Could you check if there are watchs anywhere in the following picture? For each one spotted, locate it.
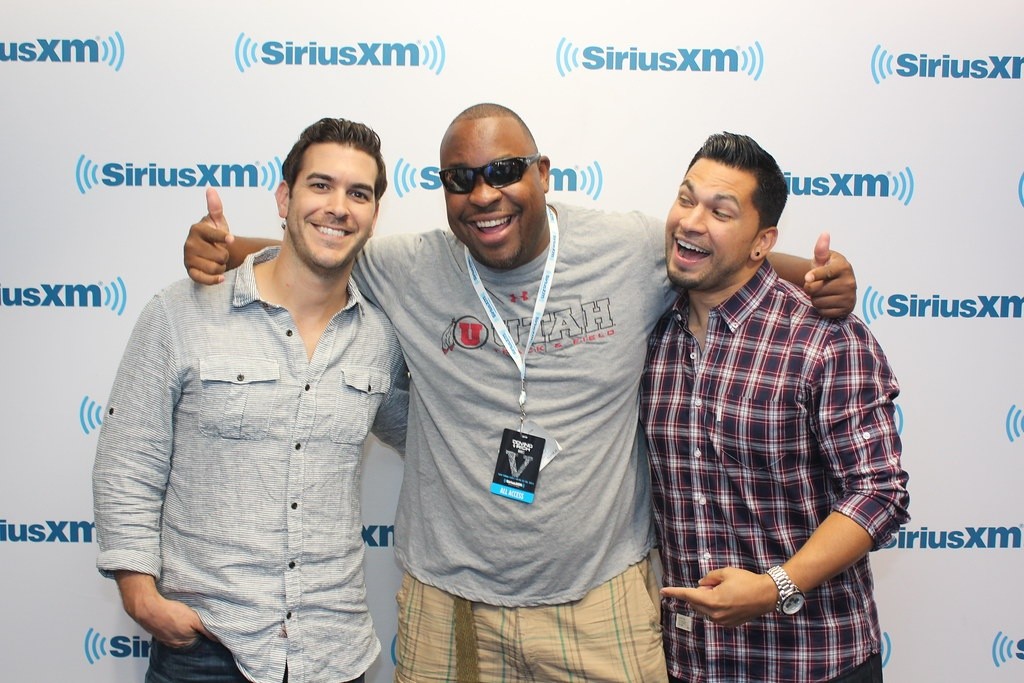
[767,565,805,616]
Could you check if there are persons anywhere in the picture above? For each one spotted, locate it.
[183,102,856,683]
[638,133,912,683]
[93,117,411,683]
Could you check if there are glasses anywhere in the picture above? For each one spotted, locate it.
[439,152,543,194]
